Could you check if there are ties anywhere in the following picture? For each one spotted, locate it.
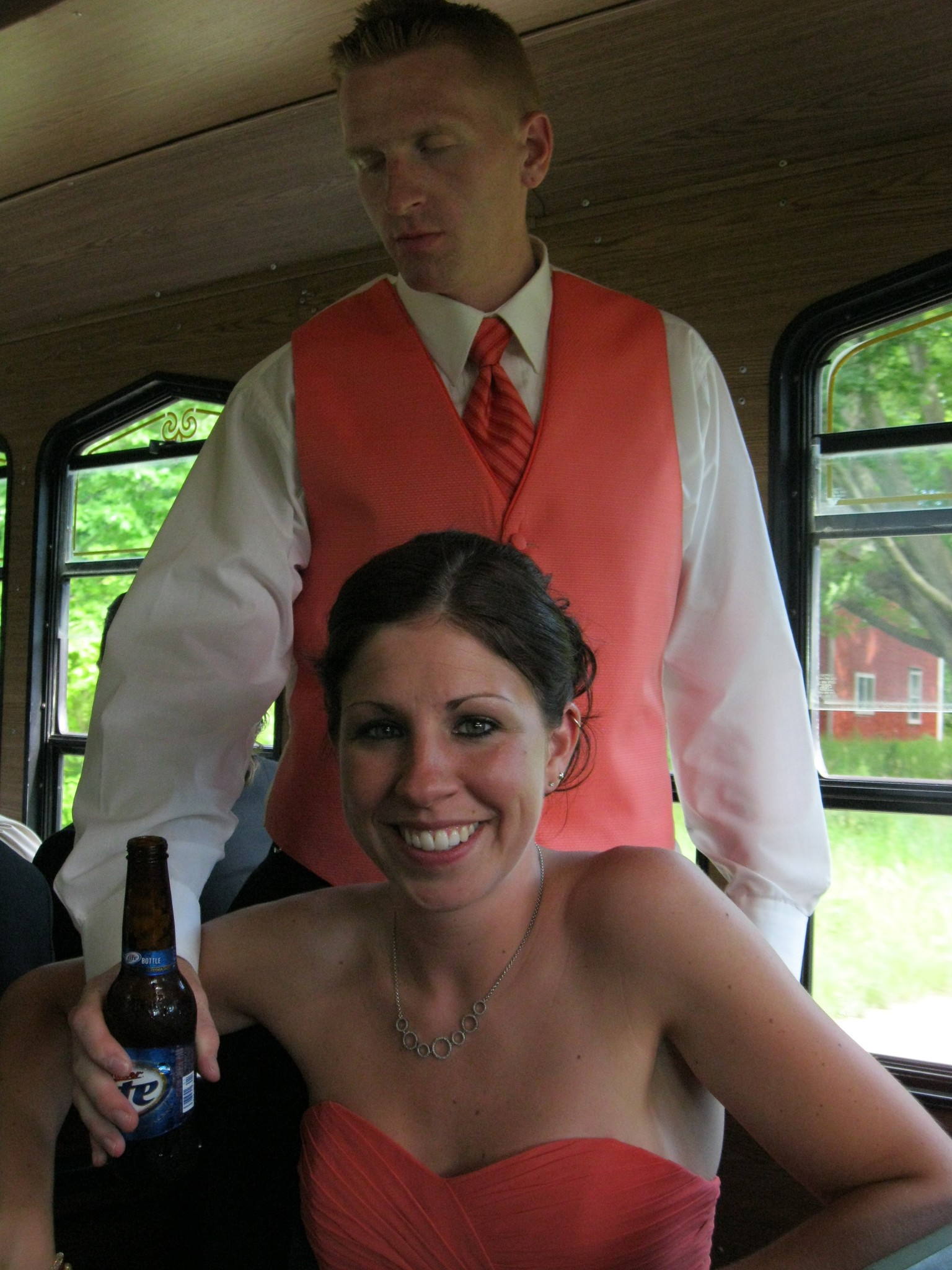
[461,318,538,502]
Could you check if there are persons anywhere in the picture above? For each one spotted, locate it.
[1,524,952,1270]
[47,1,828,1165]
[94,594,283,937]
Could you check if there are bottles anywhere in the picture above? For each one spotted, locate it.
[100,836,200,1174]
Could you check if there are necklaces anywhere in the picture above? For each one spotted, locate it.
[391,840,545,1061]
